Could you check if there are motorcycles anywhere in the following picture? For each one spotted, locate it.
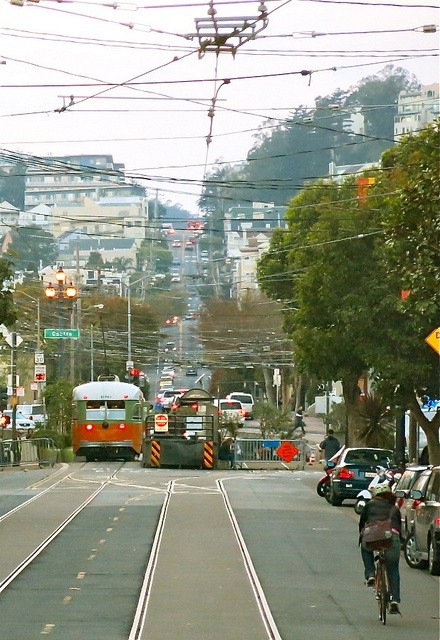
[317,460,336,497]
[354,453,403,515]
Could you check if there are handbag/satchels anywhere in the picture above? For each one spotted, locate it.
[361,520,395,551]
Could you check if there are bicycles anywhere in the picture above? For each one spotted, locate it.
[368,551,403,625]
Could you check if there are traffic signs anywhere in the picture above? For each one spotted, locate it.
[44,329,80,339]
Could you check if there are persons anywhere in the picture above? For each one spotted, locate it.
[288,394,296,411]
[218,438,237,470]
[26,428,33,439]
[359,484,401,615]
[319,429,340,464]
[292,403,306,434]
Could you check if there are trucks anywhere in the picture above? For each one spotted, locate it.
[161,223,172,234]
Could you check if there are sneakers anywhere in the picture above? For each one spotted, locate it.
[388,601,398,614]
[366,573,376,587]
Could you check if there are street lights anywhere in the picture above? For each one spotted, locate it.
[127,274,166,383]
[46,267,77,432]
[77,304,105,385]
[2,288,40,404]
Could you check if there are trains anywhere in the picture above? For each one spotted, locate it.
[73,374,152,461]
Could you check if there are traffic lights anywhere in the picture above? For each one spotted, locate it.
[129,369,139,380]
[1,416,10,428]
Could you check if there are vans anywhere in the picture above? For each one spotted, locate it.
[16,404,48,426]
[160,389,190,410]
[226,392,254,420]
[214,399,244,429]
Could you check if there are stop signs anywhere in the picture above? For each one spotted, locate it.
[154,414,168,433]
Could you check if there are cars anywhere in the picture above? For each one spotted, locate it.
[186,370,197,375]
[172,240,181,247]
[157,388,174,403]
[393,466,432,550]
[159,376,173,388]
[165,342,177,353]
[404,466,440,576]
[125,372,150,398]
[173,258,180,264]
[171,273,181,282]
[161,369,175,376]
[1,410,37,430]
[185,242,193,249]
[186,313,196,320]
[192,231,199,237]
[327,448,404,506]
[200,250,208,256]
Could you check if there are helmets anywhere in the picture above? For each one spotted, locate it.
[373,483,391,497]
